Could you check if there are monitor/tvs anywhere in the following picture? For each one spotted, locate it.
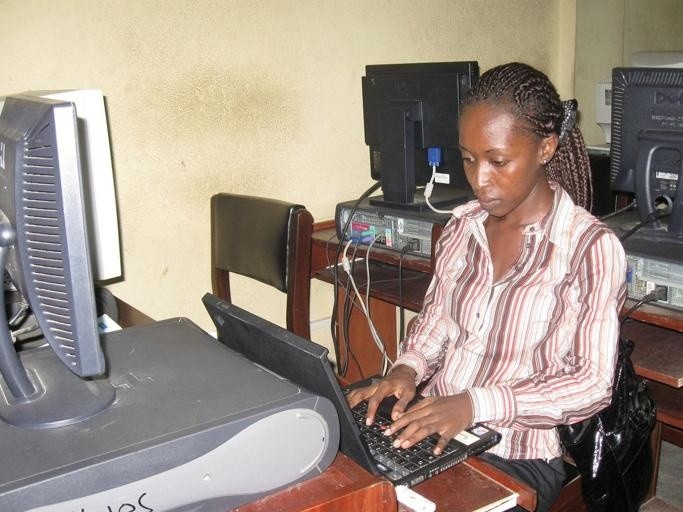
[361,61,482,214]
[608,67,683,244]
[0,93,116,428]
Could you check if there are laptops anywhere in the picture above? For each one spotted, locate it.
[201,292,503,487]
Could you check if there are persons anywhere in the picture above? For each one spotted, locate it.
[343,62,627,511]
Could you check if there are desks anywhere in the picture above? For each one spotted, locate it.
[1,283,540,512]
[309,220,682,450]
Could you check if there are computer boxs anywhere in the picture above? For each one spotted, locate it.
[0,315,340,511]
[601,210,683,315]
[334,193,474,261]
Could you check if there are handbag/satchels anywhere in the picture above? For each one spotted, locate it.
[580,340,657,512]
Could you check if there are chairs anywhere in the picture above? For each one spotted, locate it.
[210,193,314,342]
